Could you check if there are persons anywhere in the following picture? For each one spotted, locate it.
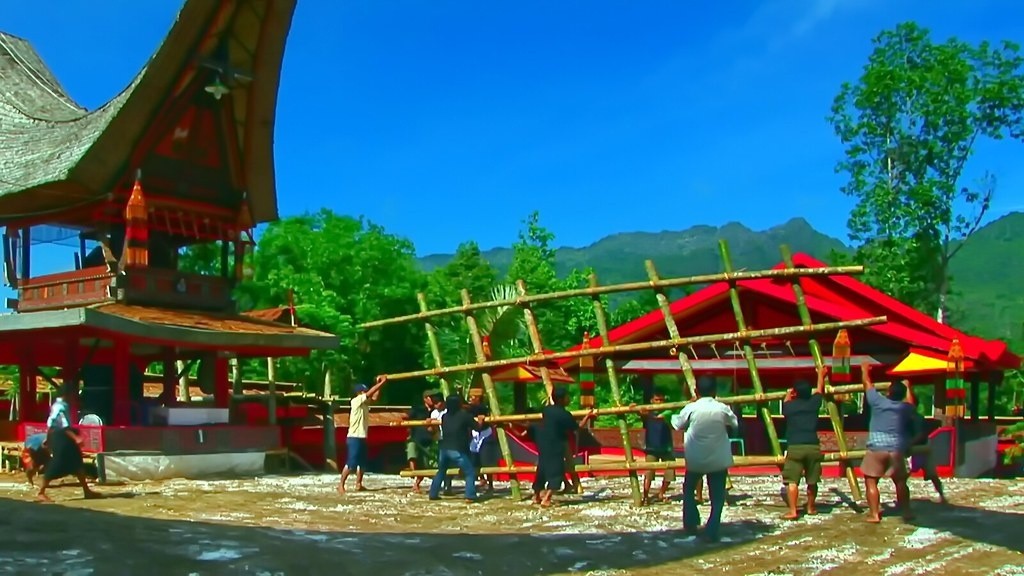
[861,360,949,524]
[629,391,677,507]
[337,374,387,493]
[780,364,829,518]
[38,383,103,503]
[531,388,599,505]
[671,377,738,540]
[395,388,495,501]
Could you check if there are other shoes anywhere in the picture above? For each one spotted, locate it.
[477,479,487,486]
[355,486,366,491]
[542,503,554,508]
[533,498,542,504]
[641,496,649,506]
[336,486,345,493]
[432,497,441,500]
[654,493,672,503]
[412,487,422,494]
[465,498,475,503]
[84,492,102,499]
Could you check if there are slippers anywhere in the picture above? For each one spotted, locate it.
[807,511,818,515]
[778,512,797,521]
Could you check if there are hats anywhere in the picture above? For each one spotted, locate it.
[423,390,431,397]
[469,388,484,396]
[353,384,368,392]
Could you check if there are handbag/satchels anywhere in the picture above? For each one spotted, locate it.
[36,493,55,502]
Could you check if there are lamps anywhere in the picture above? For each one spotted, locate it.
[203,73,229,98]
